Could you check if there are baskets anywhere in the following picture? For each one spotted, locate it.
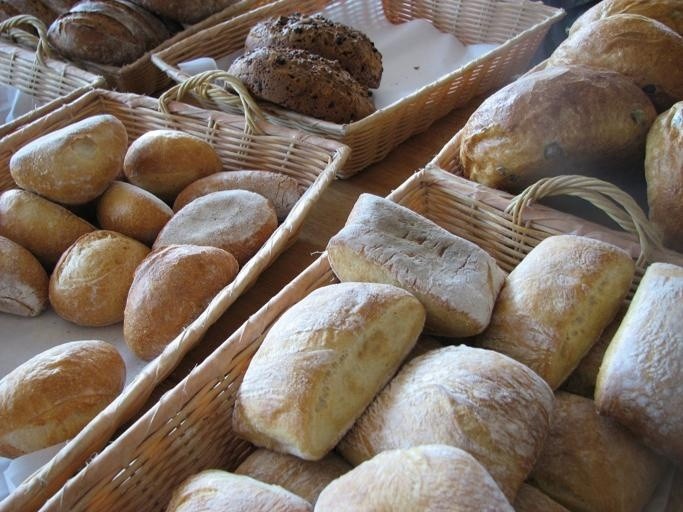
[423,60,682,266]
[0,45,105,137]
[1,1,683,511]
[37,166,678,510]
[0,69,353,511]
[151,0,568,182]
[0,0,255,98]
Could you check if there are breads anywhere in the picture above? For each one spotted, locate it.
[244,12,384,89]
[0,0,239,68]
[0,340,126,458]
[161,191,682,512]
[224,47,375,126]
[0,113,302,359]
[461,0,683,249]
